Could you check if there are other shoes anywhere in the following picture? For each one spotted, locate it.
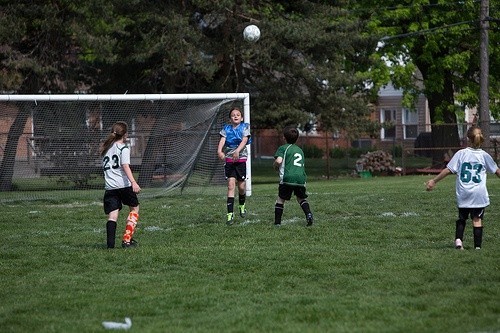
[455,239,463,248]
[475,247,481,250]
[121,238,138,248]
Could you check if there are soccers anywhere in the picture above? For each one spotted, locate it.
[243,24,261,42]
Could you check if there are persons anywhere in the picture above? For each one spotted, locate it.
[424,126,500,250]
[100,121,143,249]
[273,126,313,226]
[217,107,252,226]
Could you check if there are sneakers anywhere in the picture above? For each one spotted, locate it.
[239,204,249,220]
[305,213,314,227]
[226,213,235,226]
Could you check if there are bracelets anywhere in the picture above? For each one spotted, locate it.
[131,180,135,183]
